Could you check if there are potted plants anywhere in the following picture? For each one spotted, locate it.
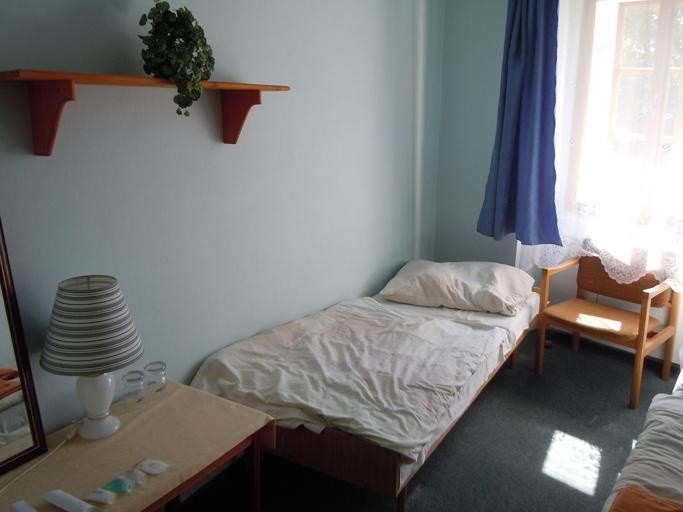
[135,1,215,115]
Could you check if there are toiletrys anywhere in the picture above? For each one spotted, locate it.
[83,468,146,505]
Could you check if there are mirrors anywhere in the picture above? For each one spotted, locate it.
[0,217,49,476]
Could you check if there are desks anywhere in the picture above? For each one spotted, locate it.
[0,380,281,512]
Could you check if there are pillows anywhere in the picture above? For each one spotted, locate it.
[378,255,535,317]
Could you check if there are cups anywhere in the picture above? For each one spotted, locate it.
[143,361,168,395]
[121,370,146,406]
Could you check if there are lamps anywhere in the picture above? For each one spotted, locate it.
[35,275,144,442]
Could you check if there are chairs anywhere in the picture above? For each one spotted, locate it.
[532,234,679,409]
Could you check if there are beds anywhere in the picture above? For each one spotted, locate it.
[189,286,545,511]
[599,372,682,511]
